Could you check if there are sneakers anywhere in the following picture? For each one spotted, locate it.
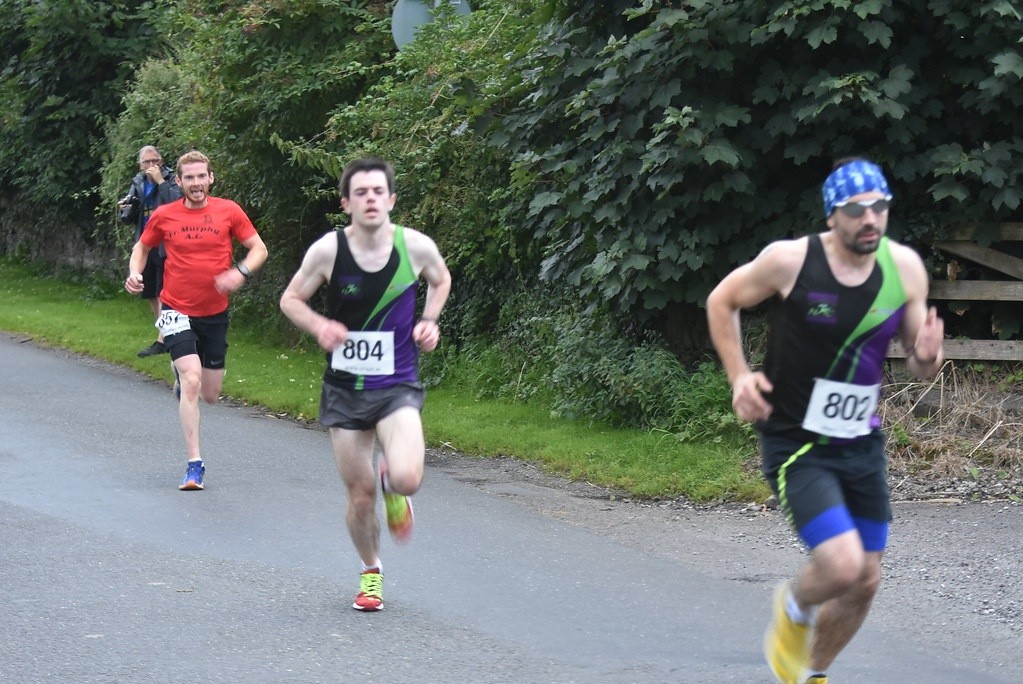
[353,563,385,612]
[798,667,829,684]
[171,363,182,401]
[177,460,205,490]
[374,455,414,542]
[764,580,808,684]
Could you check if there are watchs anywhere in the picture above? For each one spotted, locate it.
[235,263,254,284]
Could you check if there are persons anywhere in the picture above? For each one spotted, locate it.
[122,149,268,491]
[280,158,453,613]
[704,156,947,684]
[118,145,184,357]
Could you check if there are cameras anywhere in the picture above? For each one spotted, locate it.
[121,195,141,225]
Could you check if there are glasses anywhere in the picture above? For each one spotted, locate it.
[140,158,161,165]
[831,197,891,217]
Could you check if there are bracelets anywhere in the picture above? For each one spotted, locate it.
[910,344,939,367]
[420,317,435,323]
